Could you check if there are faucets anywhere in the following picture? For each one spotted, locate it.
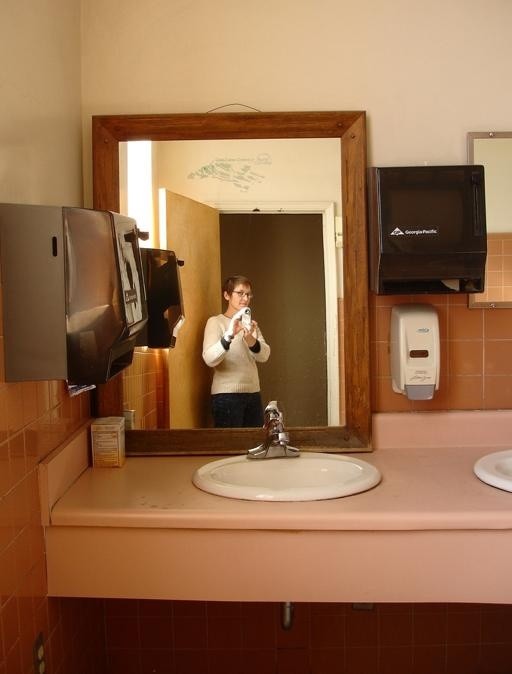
[246,402,299,459]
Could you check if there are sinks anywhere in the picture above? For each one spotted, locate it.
[474,449,512,493]
[191,452,382,502]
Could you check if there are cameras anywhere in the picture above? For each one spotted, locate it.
[242,307,251,328]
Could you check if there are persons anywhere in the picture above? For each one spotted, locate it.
[202,274,272,427]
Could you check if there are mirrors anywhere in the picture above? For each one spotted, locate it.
[467,131,512,308]
[91,111,373,457]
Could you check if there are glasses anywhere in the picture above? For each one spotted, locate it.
[232,291,253,298]
